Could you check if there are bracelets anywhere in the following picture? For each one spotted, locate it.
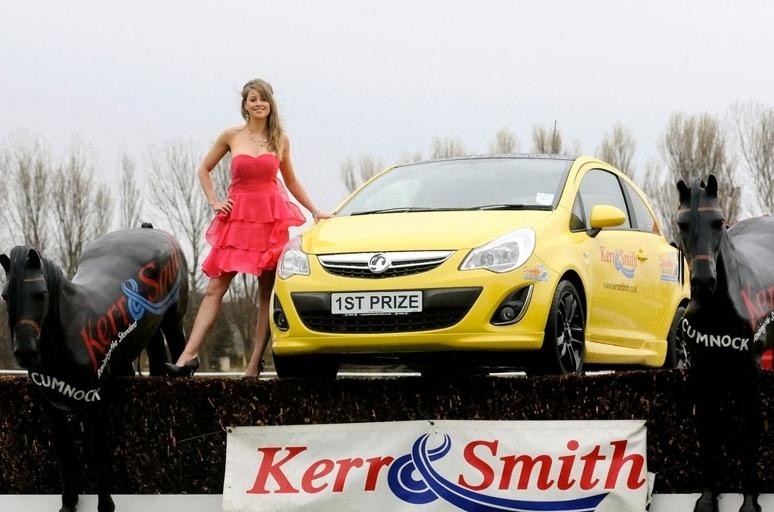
[312,209,320,217]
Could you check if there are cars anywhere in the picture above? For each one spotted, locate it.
[266,154,687,379]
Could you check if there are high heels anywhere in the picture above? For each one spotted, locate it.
[239,359,265,382]
[164,354,200,379]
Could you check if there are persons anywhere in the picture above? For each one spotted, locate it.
[165,79,332,381]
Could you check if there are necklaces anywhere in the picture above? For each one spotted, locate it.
[248,130,267,147]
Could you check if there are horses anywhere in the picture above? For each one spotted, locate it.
[672,172,774,512]
[0,221,191,512]
